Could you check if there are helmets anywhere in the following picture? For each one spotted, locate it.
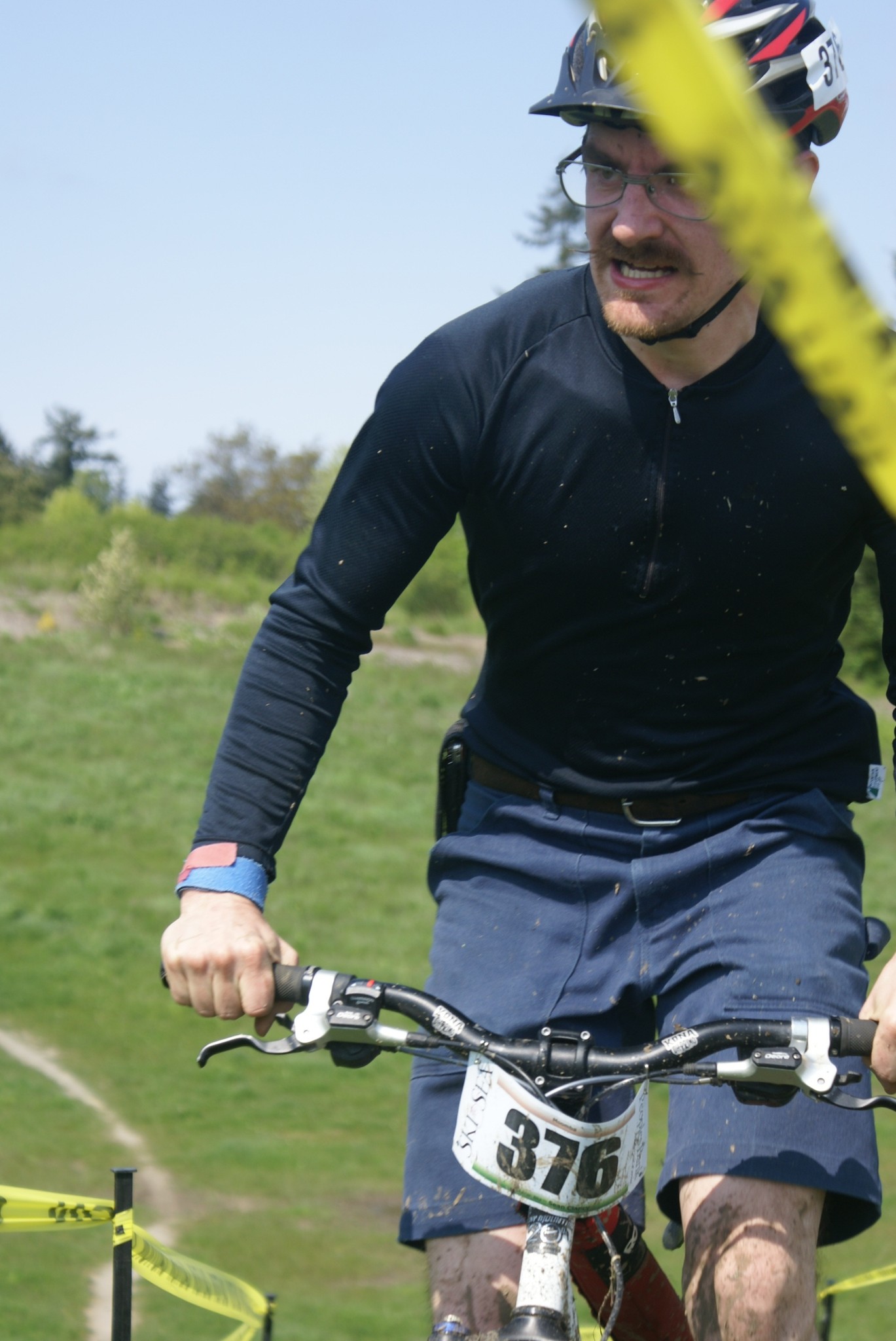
[527,0,851,146]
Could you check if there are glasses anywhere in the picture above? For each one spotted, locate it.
[554,149,724,221]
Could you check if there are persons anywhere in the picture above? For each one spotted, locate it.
[158,0,896,1341]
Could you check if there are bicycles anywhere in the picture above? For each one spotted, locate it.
[161,959,896,1341]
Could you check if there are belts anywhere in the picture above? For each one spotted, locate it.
[463,752,757,829]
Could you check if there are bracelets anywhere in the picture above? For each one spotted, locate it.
[175,842,268,912]
